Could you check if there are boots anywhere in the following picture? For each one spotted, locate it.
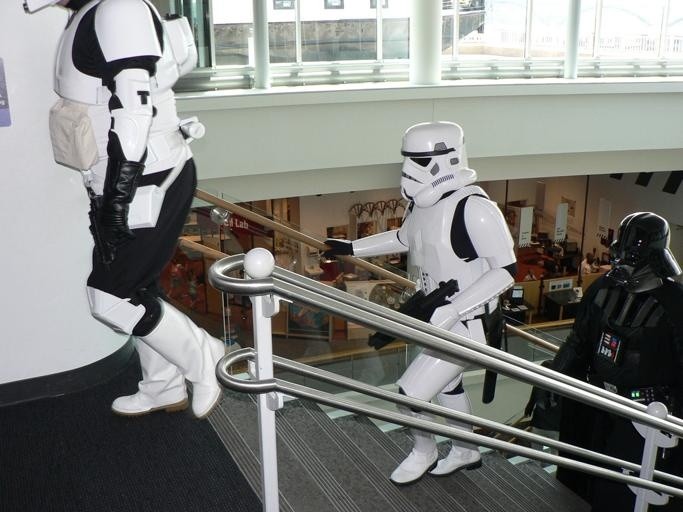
[389,392,481,485]
[111,297,225,419]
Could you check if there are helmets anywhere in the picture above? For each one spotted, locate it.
[606,212,682,293]
[401,122,476,207]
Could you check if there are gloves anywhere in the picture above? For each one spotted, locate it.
[100,161,145,244]
[319,239,353,260]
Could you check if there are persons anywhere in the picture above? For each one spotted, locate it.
[17,0,226,424]
[185,268,200,312]
[523,358,567,467]
[523,269,538,281]
[583,256,593,274]
[320,118,518,487]
[165,258,188,300]
[542,210,682,511]
[576,251,592,281]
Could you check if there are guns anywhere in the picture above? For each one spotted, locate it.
[368,279,459,351]
[90,193,126,269]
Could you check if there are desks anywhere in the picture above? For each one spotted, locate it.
[542,289,582,321]
[505,297,535,323]
